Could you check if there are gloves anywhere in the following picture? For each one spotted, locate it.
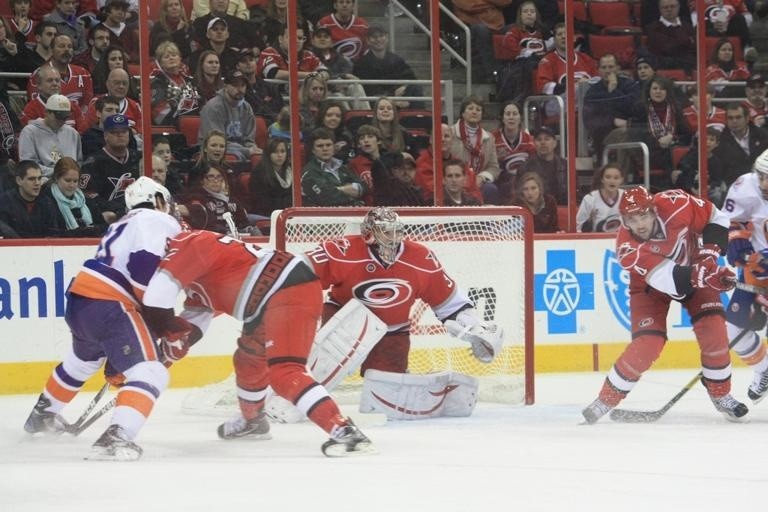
[152,318,194,361]
[690,221,768,291]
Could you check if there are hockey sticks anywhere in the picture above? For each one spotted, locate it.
[612,310,757,424]
[57,358,169,436]
[54,382,109,430]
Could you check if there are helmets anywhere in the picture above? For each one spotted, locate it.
[124,176,171,211]
[755,149,768,175]
[620,186,656,229]
[360,206,404,264]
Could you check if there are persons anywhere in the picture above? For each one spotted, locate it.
[295,206,506,416]
[717,147,767,400]
[0,2,768,237]
[579,186,749,420]
[20,175,184,462]
[103,222,375,456]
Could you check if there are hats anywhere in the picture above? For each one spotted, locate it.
[226,70,248,83]
[46,95,71,118]
[207,17,228,31]
[104,114,127,129]
